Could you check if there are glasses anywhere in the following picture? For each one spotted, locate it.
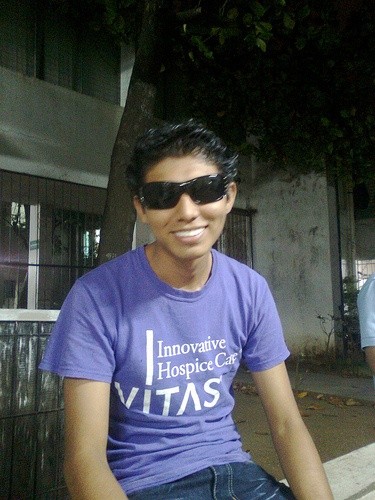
[139,170,229,211]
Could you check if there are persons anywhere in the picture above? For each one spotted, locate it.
[38,120,340,500]
[356,270,375,381]
